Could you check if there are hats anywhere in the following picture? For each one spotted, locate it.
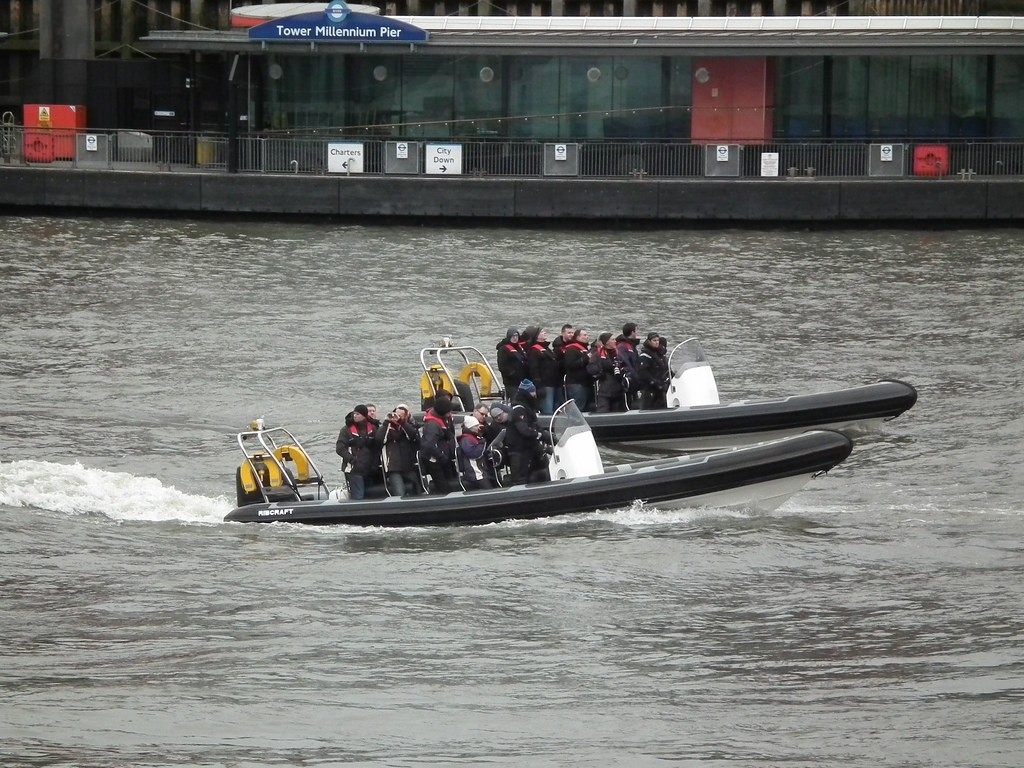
[519,379,535,393]
[354,404,368,418]
[599,333,613,345]
[529,327,543,340]
[491,407,503,418]
[507,328,519,339]
[464,416,479,429]
[434,398,451,415]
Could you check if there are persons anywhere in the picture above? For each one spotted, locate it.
[494,320,680,419]
[335,378,559,499]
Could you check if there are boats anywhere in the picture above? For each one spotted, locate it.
[411,336,917,449]
[224,398,853,527]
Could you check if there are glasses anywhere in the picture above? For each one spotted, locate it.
[477,410,488,417]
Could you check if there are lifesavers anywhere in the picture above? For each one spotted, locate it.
[459,362,493,397]
[421,364,455,399]
[271,444,312,483]
[240,454,283,494]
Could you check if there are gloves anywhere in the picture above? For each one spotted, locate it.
[537,388,546,399]
[439,454,448,465]
[479,438,486,445]
[350,457,356,464]
